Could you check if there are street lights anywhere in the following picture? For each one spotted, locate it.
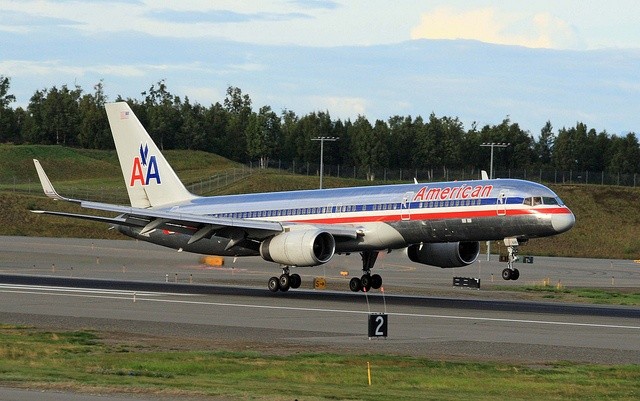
[309,133,343,189]
[480,138,511,180]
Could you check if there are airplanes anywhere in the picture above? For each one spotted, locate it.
[25,99,578,295]
